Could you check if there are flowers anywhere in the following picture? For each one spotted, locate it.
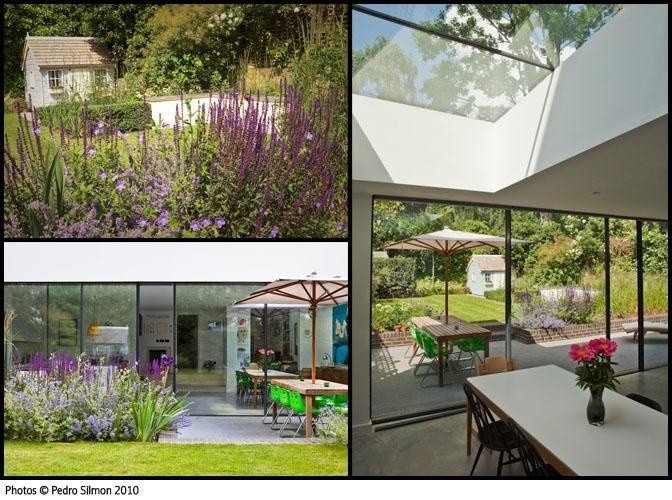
[568,338,621,398]
[255,347,275,368]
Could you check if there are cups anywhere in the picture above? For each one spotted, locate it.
[455,325,459,329]
[324,382,329,387]
[300,376,304,381]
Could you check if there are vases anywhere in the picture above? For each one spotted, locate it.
[262,362,268,373]
[587,385,605,426]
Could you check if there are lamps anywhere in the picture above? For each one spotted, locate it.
[86,283,100,337]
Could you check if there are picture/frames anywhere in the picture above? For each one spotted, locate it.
[59,319,77,347]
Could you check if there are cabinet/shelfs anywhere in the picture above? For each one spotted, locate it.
[301,368,348,385]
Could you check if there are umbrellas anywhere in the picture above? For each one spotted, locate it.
[234,272,348,384]
[379,225,534,324]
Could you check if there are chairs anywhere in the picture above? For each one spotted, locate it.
[405,314,491,387]
[477,356,518,377]
[625,393,661,413]
[505,417,563,477]
[462,383,537,476]
[236,367,349,439]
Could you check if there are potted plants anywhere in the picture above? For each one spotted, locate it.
[203,360,216,374]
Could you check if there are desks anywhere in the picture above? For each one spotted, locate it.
[88,361,121,390]
[465,365,669,476]
[15,367,48,385]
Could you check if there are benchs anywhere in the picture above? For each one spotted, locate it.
[622,321,668,343]
